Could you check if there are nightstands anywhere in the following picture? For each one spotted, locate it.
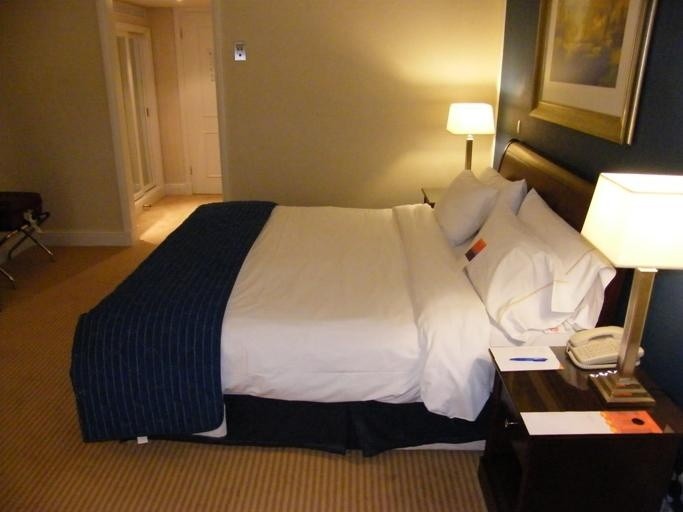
[421,186,446,208]
[478,349,683,512]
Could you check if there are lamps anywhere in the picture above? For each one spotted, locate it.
[580,173,683,409]
[446,102,497,169]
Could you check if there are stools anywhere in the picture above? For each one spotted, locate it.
[0,192,57,288]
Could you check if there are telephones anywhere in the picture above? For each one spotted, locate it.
[565,326,645,370]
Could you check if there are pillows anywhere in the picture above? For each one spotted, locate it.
[433,167,617,347]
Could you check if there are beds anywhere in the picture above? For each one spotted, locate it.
[70,138,635,457]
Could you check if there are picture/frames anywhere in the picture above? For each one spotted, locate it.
[528,0,659,147]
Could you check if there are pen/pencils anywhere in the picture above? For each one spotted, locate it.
[510,358,548,362]
